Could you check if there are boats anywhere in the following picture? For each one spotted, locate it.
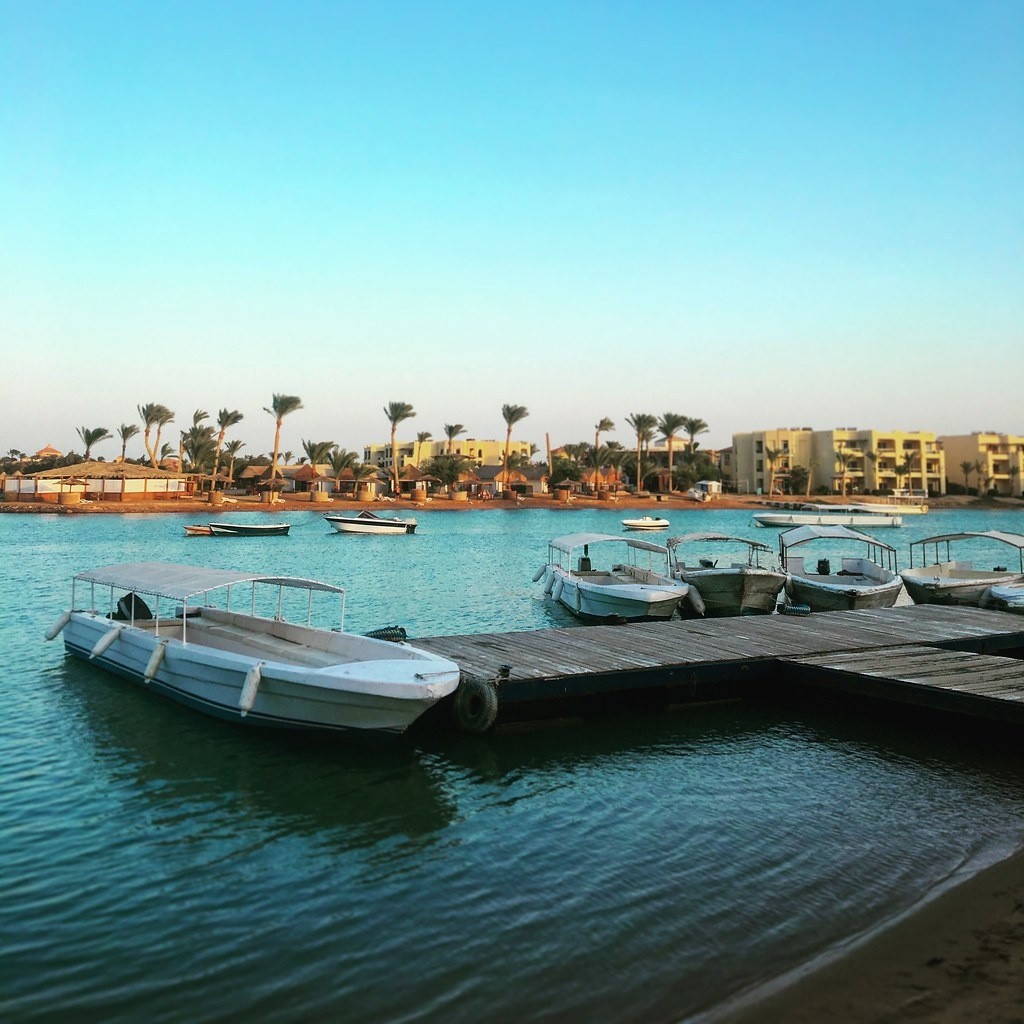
[666,533,785,617]
[850,495,929,514]
[60,561,460,738]
[323,510,417,535]
[978,580,1024,614]
[752,504,903,528]
[899,530,1024,607]
[182,525,212,535]
[543,532,690,623]
[207,522,291,536]
[620,518,669,530]
[778,523,904,611]
